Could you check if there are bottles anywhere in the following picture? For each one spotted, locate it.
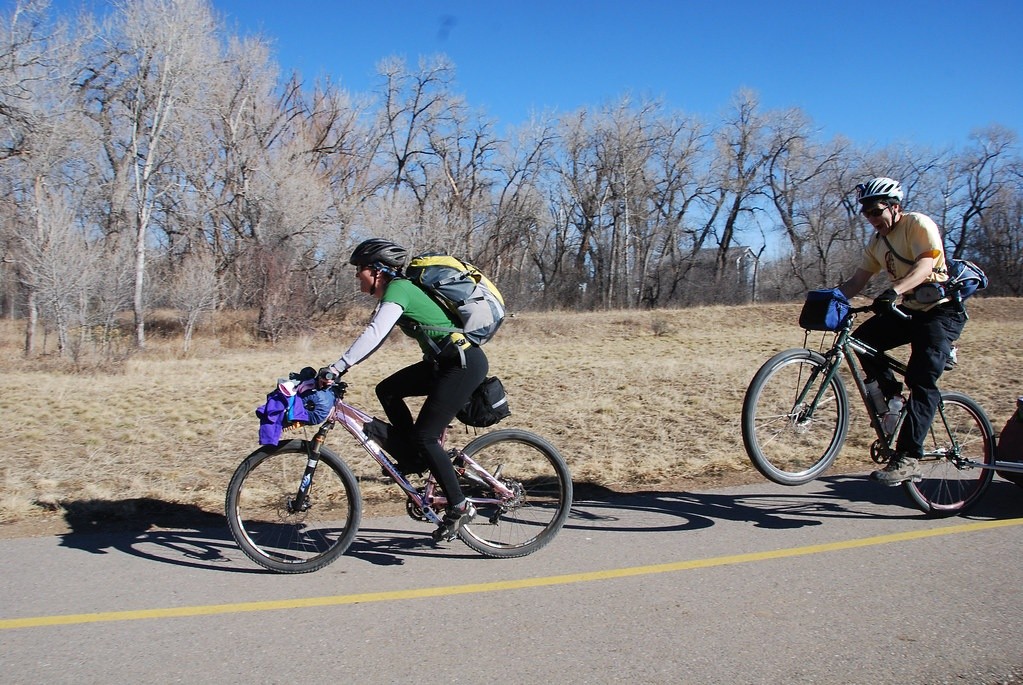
[863,377,889,415]
[880,394,904,436]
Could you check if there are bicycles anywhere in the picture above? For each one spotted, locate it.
[739,287,997,518]
[224,366,574,575]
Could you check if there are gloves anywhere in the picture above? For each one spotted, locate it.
[872,288,898,317]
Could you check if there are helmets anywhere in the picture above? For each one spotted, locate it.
[349,237,408,268]
[855,177,904,201]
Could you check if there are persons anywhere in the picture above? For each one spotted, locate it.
[314,238,489,539]
[837,177,964,485]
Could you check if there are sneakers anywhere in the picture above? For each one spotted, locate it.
[432,500,478,539]
[869,456,922,486]
[382,464,420,476]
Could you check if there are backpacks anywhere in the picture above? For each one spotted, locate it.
[405,246,505,347]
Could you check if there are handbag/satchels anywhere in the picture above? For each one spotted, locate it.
[945,256,989,299]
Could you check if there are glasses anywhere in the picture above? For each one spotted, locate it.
[356,265,366,274]
[862,206,890,218]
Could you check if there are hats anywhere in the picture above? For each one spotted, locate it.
[858,195,902,216]
